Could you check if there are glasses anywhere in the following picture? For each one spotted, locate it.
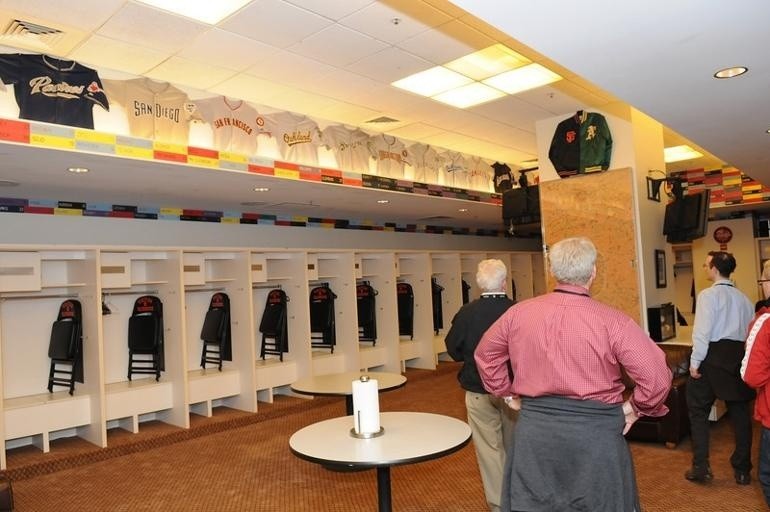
[702,263,714,267]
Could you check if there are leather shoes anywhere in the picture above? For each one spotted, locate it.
[685,466,711,481]
[736,470,751,484]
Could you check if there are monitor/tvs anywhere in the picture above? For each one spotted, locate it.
[662,189,711,244]
[647,303,676,343]
[502,185,541,225]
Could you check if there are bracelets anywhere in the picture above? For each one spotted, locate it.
[504,396,513,404]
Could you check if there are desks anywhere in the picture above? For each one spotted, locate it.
[287,370,473,510]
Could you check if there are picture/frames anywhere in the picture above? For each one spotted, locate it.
[653,249,667,288]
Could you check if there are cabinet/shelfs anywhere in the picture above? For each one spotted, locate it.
[0,241,543,451]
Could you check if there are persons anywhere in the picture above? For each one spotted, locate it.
[444,257,520,511]
[740,260,770,510]
[474,236,673,512]
[683,250,754,483]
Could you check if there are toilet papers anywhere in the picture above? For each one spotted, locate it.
[351,378,381,435]
